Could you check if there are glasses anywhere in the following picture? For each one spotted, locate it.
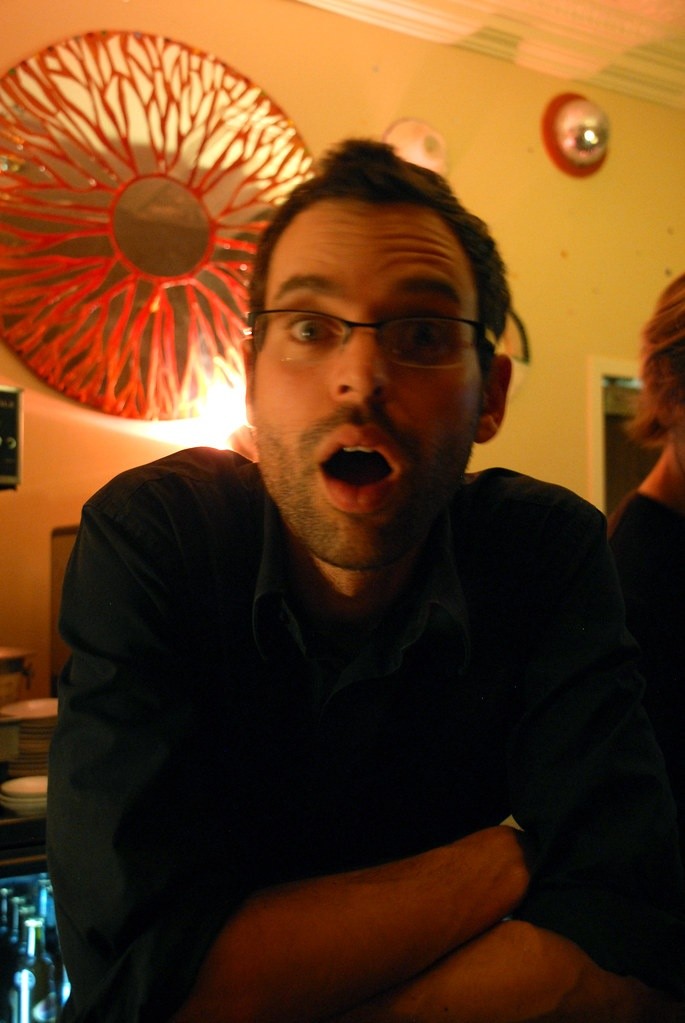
[247,308,488,368]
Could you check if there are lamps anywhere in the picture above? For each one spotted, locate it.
[380,118,453,182]
[539,92,609,179]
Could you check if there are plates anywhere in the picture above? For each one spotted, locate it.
[0,776,49,818]
[0,698,58,775]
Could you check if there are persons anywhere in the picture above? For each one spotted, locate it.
[46,137,685,1023]
[605,271,684,858]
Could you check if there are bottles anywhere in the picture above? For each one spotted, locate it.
[0,873,65,1023]
